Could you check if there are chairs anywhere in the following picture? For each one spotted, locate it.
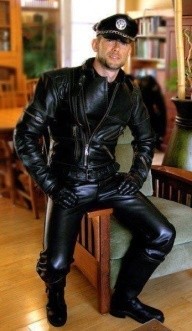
[0,75,48,219]
[73,126,192,313]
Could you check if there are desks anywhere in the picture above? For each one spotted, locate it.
[0,106,23,141]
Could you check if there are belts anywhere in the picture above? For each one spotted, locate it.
[66,163,120,183]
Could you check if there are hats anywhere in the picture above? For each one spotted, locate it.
[93,13,139,43]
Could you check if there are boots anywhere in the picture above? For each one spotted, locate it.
[45,277,67,327]
[110,252,165,324]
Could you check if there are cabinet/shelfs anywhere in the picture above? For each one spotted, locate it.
[0,0,23,93]
[128,16,192,99]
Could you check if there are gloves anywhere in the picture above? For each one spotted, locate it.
[118,171,143,195]
[50,184,80,209]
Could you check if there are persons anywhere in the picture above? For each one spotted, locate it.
[14,14,176,328]
[125,74,177,156]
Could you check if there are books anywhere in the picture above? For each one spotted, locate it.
[132,16,176,94]
